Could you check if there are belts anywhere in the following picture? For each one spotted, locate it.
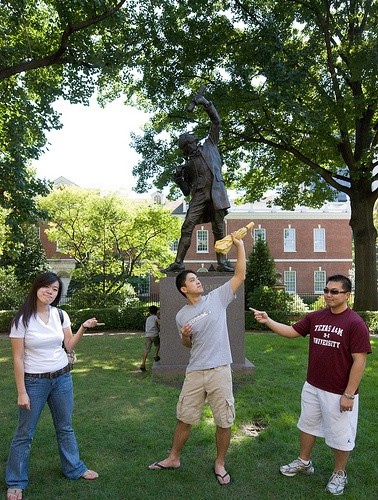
[25,364,71,379]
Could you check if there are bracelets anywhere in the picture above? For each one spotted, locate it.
[343,393,354,400]
[82,323,85,329]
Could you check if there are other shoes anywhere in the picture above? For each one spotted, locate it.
[139,365,146,371]
[154,356,160,361]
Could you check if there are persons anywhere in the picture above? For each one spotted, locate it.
[7,273,105,500]
[149,230,246,485]
[140,306,160,371]
[250,275,373,493]
[160,96,235,273]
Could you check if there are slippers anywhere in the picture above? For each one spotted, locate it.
[147,462,181,470]
[6,489,24,500]
[82,470,99,479]
[212,467,232,486]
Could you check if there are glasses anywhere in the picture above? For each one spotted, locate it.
[323,288,346,294]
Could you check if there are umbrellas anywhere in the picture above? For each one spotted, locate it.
[214,221,256,254]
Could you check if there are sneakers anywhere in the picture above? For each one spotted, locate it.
[325,470,348,495]
[279,459,315,477]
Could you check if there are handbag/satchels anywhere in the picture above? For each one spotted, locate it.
[58,309,77,365]
